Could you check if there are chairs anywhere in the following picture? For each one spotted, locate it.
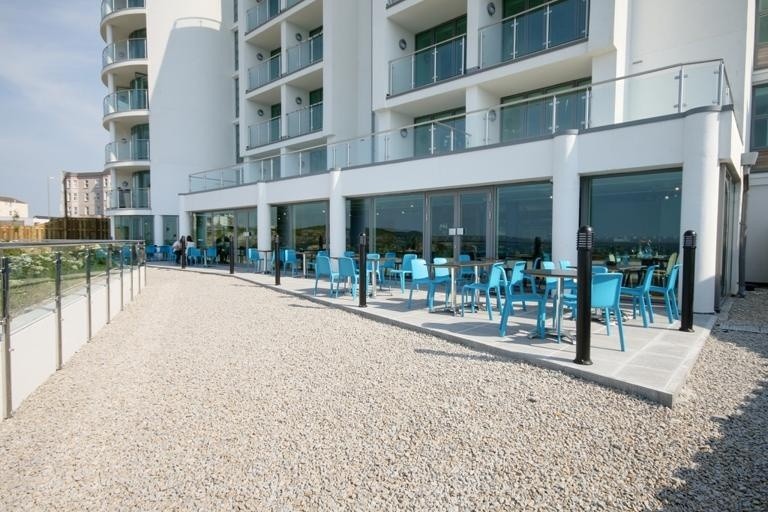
[495,253,628,354]
[144,242,223,269]
[632,263,684,324]
[247,243,313,278]
[314,247,527,319]
[604,264,660,327]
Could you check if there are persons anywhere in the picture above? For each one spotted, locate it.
[186,235,195,265]
[172,235,185,264]
[216,232,229,264]
